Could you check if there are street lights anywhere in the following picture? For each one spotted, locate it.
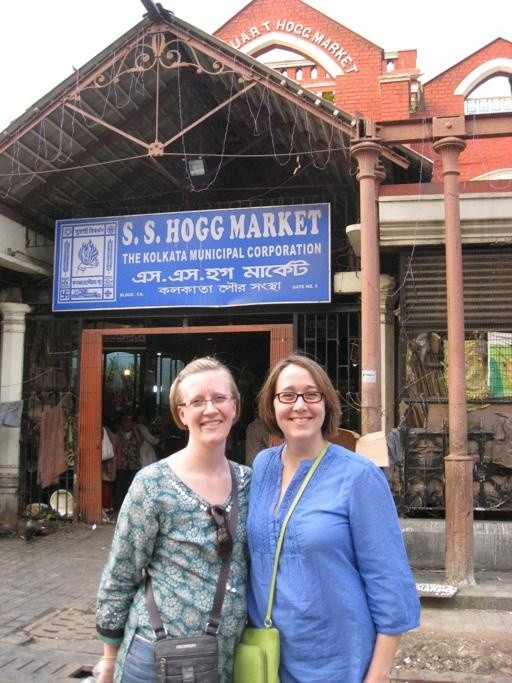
[230,623,283,682]
[154,633,222,683]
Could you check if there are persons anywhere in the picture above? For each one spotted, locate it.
[244,355,422,683]
[105,404,169,501]
[94,355,257,683]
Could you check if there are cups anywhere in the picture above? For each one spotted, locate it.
[173,393,240,411]
[274,390,325,404]
[210,503,234,561]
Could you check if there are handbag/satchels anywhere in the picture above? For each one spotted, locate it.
[102,654,117,659]
